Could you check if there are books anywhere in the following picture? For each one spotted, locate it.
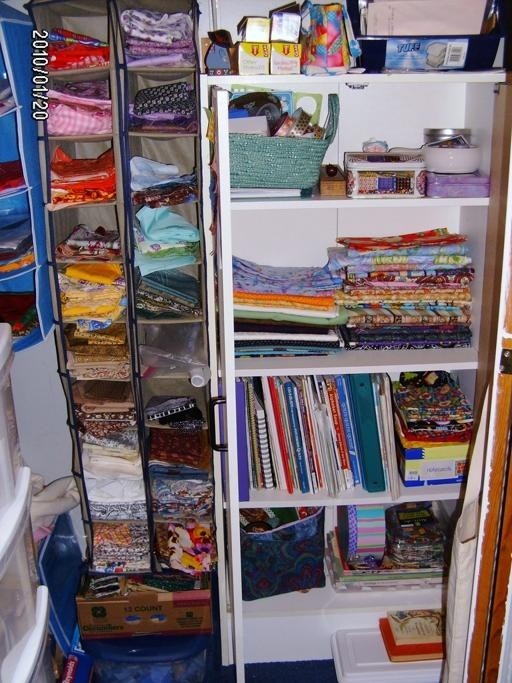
[234,374,403,504]
[377,609,452,667]
[324,527,445,591]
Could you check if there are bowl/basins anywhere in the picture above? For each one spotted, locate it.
[425,144,482,173]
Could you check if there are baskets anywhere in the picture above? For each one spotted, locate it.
[230,95,339,189]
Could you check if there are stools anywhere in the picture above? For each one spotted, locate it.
[81,630,213,682]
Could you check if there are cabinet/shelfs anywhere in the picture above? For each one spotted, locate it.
[22,0,227,585]
[195,68,512,683]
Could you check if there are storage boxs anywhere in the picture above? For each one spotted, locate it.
[427,166,490,198]
[390,386,474,489]
[240,507,327,605]
[1,321,51,681]
[359,0,495,37]
[77,571,213,641]
[341,151,425,200]
[355,35,503,73]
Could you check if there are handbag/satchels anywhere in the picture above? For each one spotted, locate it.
[300,0,362,76]
